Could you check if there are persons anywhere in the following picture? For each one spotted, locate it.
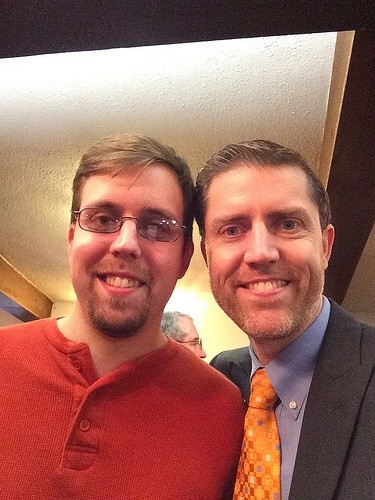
[160,312,206,361]
[0,133,245,500]
[194,139,375,500]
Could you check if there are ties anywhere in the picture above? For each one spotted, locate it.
[232,366,282,500]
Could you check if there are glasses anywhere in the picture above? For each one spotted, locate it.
[73,208,189,243]
[174,338,202,350]
